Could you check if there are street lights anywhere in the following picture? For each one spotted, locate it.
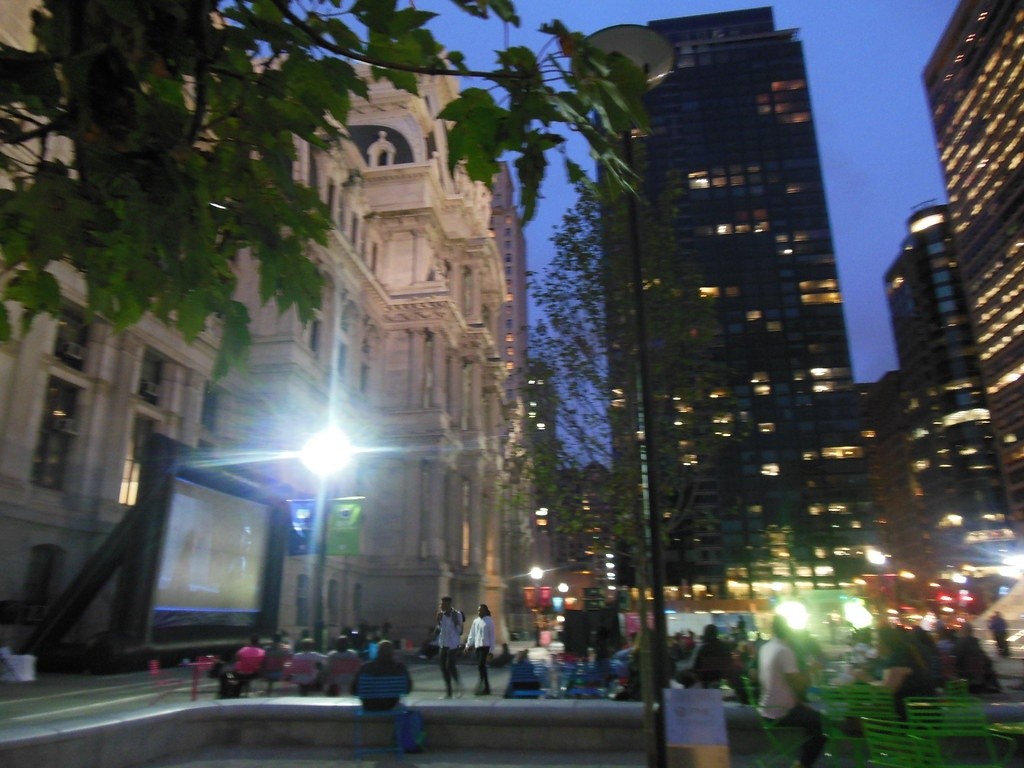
[572,23,679,768]
[298,426,359,648]
[530,563,544,613]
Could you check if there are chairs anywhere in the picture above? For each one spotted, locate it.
[351,674,409,762]
[743,674,1024,768]
[511,651,625,701]
[149,655,363,707]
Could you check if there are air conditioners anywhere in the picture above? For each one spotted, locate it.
[143,381,161,399]
[63,341,84,360]
[55,418,82,438]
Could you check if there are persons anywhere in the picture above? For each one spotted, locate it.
[433,597,463,700]
[464,604,494,695]
[234,628,414,711]
[533,610,1012,768]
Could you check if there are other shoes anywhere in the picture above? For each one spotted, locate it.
[478,688,490,695]
[456,689,462,699]
[438,695,452,699]
